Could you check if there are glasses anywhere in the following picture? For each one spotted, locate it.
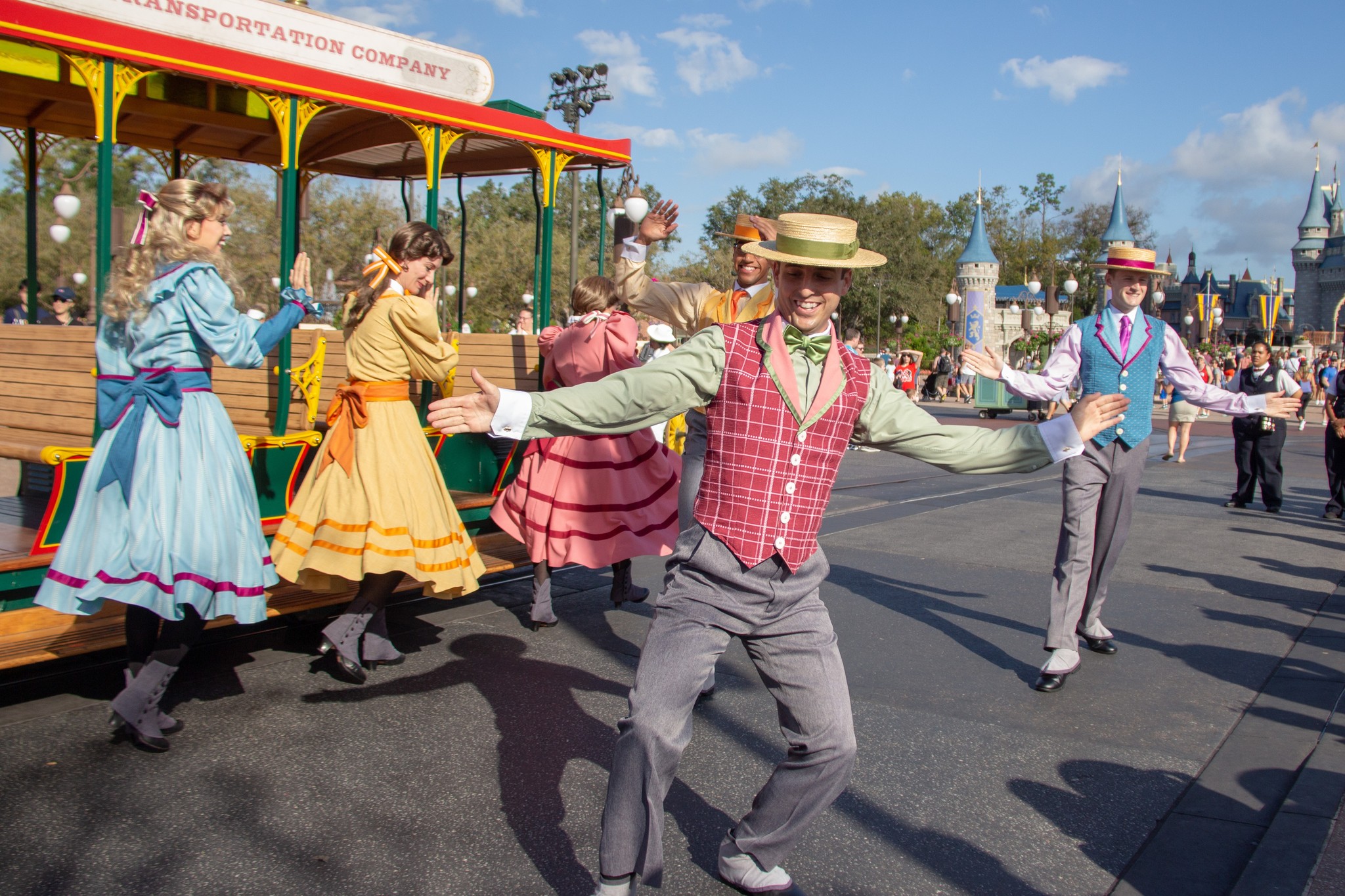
[734,242,763,259]
[902,354,911,357]
[518,316,533,321]
[52,295,69,304]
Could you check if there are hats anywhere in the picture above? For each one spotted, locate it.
[1290,352,1296,357]
[50,288,76,302]
[1196,354,1205,361]
[742,213,887,267]
[1089,246,1171,276]
[715,214,779,242]
[647,324,676,343]
[1331,356,1337,361]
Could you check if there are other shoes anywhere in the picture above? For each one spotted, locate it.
[1225,497,1343,518]
[938,394,973,404]
[1322,420,1328,425]
[1316,400,1326,406]
[1199,413,1208,418]
[719,872,807,896]
[1152,404,1168,409]
[1299,419,1305,431]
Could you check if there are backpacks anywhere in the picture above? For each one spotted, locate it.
[938,356,952,374]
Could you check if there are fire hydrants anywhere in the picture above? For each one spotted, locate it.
[544,61,615,318]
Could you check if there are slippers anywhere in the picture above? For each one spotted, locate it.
[1161,452,1187,463]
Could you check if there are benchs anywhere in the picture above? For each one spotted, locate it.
[0,323,547,558]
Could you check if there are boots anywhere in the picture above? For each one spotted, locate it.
[108,560,650,751]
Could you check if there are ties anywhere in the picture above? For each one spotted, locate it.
[731,289,748,323]
[1119,316,1132,364]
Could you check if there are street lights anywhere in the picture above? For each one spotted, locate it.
[832,301,842,341]
[1234,326,1239,356]
[1009,291,1043,371]
[1184,298,1224,353]
[50,157,100,326]
[1152,279,1165,320]
[946,277,962,369]
[890,304,909,359]
[606,164,649,313]
[1028,259,1078,359]
[1241,330,1247,346]
[521,278,534,309]
[444,269,477,327]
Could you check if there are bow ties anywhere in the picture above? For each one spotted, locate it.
[783,324,833,366]
[1253,368,1265,372]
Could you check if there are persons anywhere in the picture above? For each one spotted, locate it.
[5,279,51,324]
[873,348,923,405]
[614,200,779,699]
[490,276,683,631]
[507,308,540,335]
[32,178,325,750]
[1038,388,1074,423]
[638,324,676,366]
[1155,353,1197,464]
[962,248,1302,693]
[426,213,1130,896]
[267,221,487,680]
[1153,349,1251,418]
[843,328,864,358]
[1275,348,1345,430]
[1026,355,1041,370]
[932,342,976,404]
[1225,340,1303,513]
[1323,368,1345,519]
[40,287,83,325]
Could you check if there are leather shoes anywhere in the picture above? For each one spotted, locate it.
[1074,628,1118,654]
[1035,661,1081,692]
